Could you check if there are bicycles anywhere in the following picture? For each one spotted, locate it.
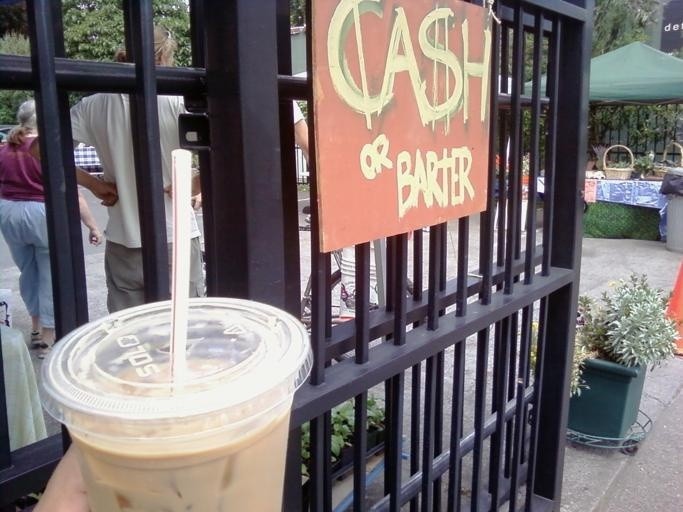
[201,205,417,364]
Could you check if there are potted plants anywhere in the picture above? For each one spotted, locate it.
[530,271,682,457]
[300,393,386,484]
[591,144,610,171]
[634,157,655,179]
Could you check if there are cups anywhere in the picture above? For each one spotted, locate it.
[37,294,314,512]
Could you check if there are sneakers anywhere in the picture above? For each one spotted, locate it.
[31,331,52,356]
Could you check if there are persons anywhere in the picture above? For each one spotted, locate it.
[191,191,203,210]
[290,91,310,172]
[29,438,96,512]
[28,27,206,315]
[585,143,598,170]
[0,99,106,359]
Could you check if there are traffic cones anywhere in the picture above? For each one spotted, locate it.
[663,260,683,358]
